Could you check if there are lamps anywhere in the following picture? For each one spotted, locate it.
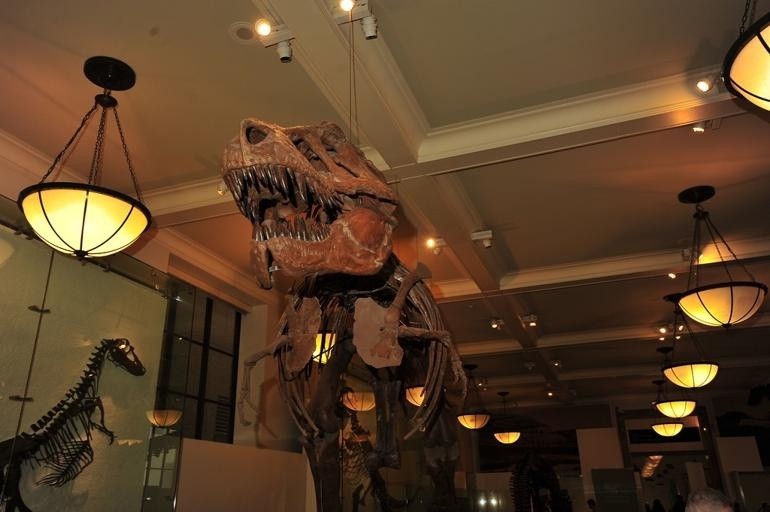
[651,185,768,437]
[334,0,378,40]
[457,367,491,429]
[340,356,375,413]
[723,1,769,114]
[407,357,448,408]
[697,72,722,96]
[489,319,502,330]
[427,237,441,256]
[144,408,182,427]
[529,316,536,327]
[693,118,721,133]
[642,454,664,478]
[17,56,152,258]
[480,230,493,248]
[255,17,294,63]
[310,314,338,366]
[494,390,521,444]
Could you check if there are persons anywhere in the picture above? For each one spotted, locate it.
[586,479,769,511]
